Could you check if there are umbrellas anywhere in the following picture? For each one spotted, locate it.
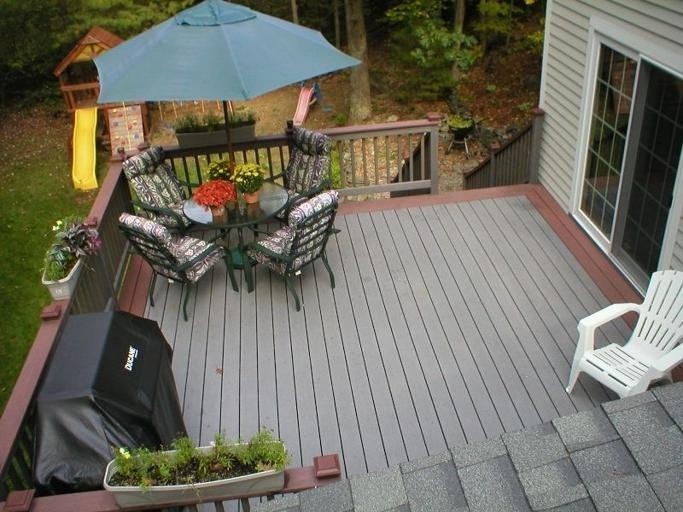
[92,0,361,208]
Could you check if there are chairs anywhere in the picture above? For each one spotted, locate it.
[252,128,333,235]
[243,190,340,312]
[122,146,227,240]
[118,212,238,321]
[565,270,683,399]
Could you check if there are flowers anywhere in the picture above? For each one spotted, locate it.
[193,181,235,208]
[205,159,230,180]
[229,164,266,193]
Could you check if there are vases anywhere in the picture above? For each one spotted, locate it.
[208,204,225,217]
[241,189,260,204]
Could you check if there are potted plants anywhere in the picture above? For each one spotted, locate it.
[173,110,257,149]
[103,426,292,508]
[41,215,101,300]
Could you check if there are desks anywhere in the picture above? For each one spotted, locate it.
[182,178,288,269]
